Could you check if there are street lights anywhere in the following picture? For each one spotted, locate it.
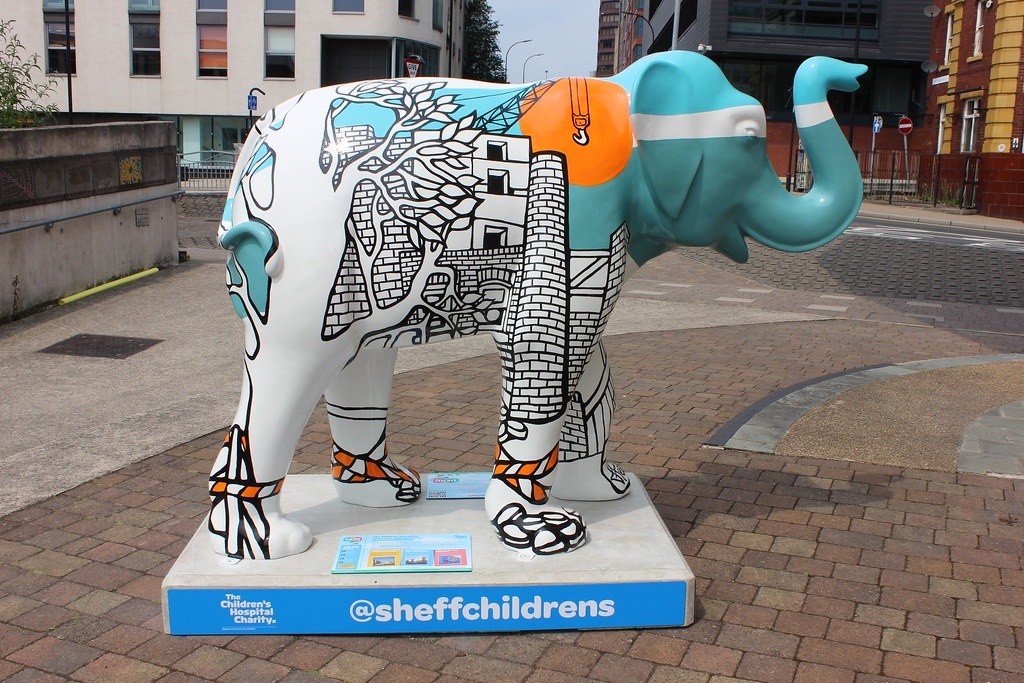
[545,70,548,79]
[505,39,532,83]
[619,10,655,53]
[523,53,544,84]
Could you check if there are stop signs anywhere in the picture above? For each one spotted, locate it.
[898,117,913,134]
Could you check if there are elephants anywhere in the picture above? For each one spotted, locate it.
[208,50,868,556]
